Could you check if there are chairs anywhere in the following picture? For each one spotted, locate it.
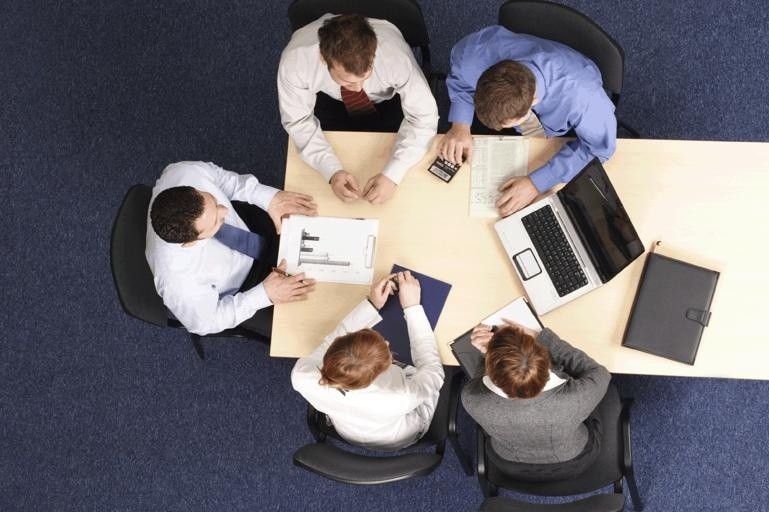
[497,0,640,139]
[292,368,474,486]
[287,0,447,85]
[476,397,642,512]
[109,184,270,360]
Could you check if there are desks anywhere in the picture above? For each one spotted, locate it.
[270,130,769,382]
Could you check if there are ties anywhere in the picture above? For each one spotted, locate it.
[340,85,377,118]
[515,106,548,139]
[214,222,265,261]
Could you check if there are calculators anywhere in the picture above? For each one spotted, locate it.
[428,149,468,183]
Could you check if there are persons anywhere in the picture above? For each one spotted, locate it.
[435,26,618,219]
[287,268,448,454]
[141,157,316,346]
[459,316,615,480]
[277,11,439,204]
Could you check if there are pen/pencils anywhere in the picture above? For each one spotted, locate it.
[272,267,310,286]
[523,298,545,331]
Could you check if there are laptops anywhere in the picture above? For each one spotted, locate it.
[494,156,645,316]
